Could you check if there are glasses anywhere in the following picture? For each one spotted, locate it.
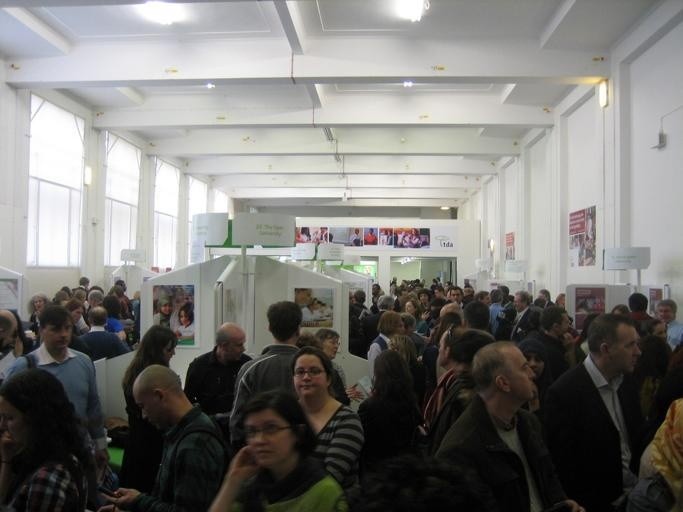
[294,369,325,377]
[447,323,456,337]
[244,424,291,437]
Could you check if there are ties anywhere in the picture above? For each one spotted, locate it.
[612,390,631,464]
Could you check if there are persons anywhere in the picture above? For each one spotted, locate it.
[175,302,193,340]
[118,326,177,495]
[295,288,333,327]
[350,454,501,512]
[1,301,110,505]
[296,227,305,243]
[0,369,96,511]
[519,338,555,421]
[542,313,647,510]
[459,301,488,329]
[295,331,323,352]
[95,365,226,512]
[313,327,365,404]
[380,229,388,245]
[230,300,335,457]
[428,338,586,512]
[421,326,466,438]
[394,228,422,248]
[313,232,319,241]
[367,311,405,397]
[425,304,462,345]
[427,330,498,456]
[388,231,393,245]
[349,277,474,311]
[398,312,425,357]
[476,286,600,380]
[356,348,424,489]
[0,276,140,380]
[153,293,172,328]
[422,312,462,397]
[301,227,311,241]
[320,227,328,241]
[349,228,361,246]
[370,296,395,338]
[609,291,682,509]
[183,322,255,447]
[364,228,377,245]
[402,301,431,338]
[207,388,351,512]
[286,343,365,507]
